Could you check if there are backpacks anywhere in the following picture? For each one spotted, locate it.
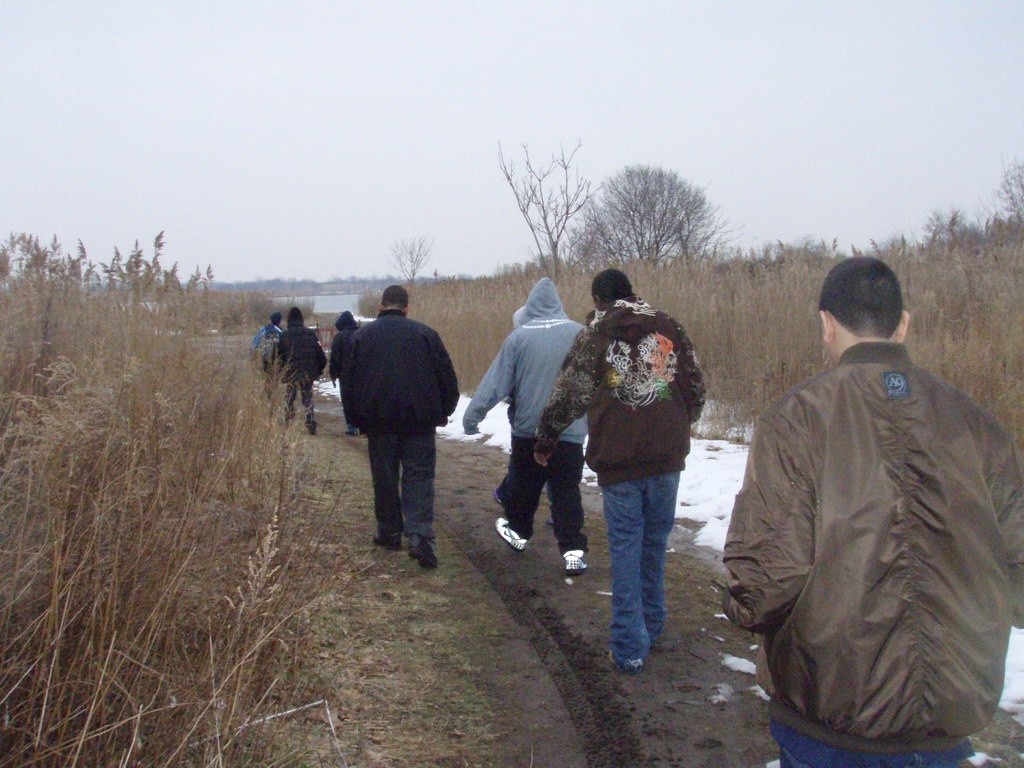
[259,326,280,365]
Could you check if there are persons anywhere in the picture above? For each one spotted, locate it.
[252,275,591,575]
[531,268,706,674]
[716,254,1024,768]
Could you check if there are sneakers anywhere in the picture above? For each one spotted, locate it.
[564,554,588,575]
[495,516,528,550]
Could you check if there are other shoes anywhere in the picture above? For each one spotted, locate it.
[406,532,440,570]
[308,426,316,435]
[346,427,359,436]
[606,649,618,667]
[372,530,404,548]
